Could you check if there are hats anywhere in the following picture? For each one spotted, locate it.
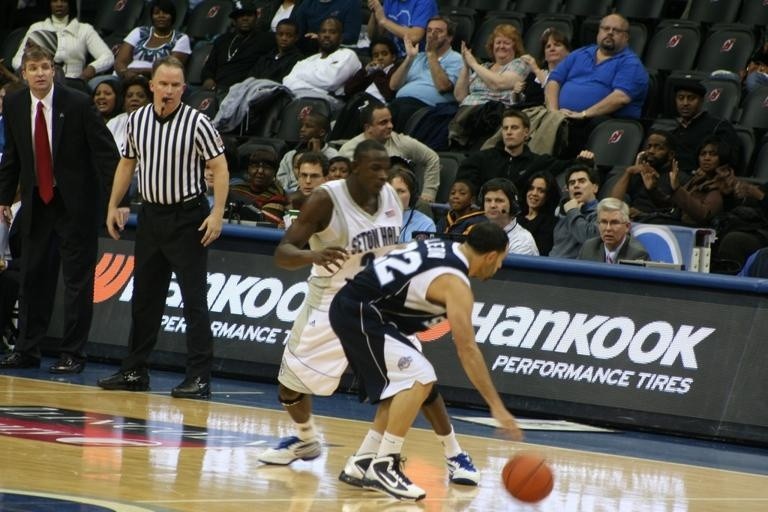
[229,1,257,18]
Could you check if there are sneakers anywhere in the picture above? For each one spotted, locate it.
[99,369,150,390]
[258,436,322,464]
[341,453,425,502]
[172,377,209,398]
[446,452,480,485]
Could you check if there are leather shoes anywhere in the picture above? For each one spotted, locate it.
[0,353,40,368]
[49,359,82,373]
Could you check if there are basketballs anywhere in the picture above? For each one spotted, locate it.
[503,453,553,502]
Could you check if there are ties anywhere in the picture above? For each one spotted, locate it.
[35,102,54,204]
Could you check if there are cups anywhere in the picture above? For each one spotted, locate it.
[289,209,300,225]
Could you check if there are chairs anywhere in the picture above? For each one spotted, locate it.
[2,0,225,120]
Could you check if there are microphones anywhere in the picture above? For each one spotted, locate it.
[502,209,510,215]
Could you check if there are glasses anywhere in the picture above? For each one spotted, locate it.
[600,24,630,34]
[594,219,629,225]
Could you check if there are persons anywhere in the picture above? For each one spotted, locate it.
[327,221,526,500]
[1,1,230,404]
[233,0,767,278]
[253,138,482,489]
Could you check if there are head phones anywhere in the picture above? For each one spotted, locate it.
[478,178,519,213]
